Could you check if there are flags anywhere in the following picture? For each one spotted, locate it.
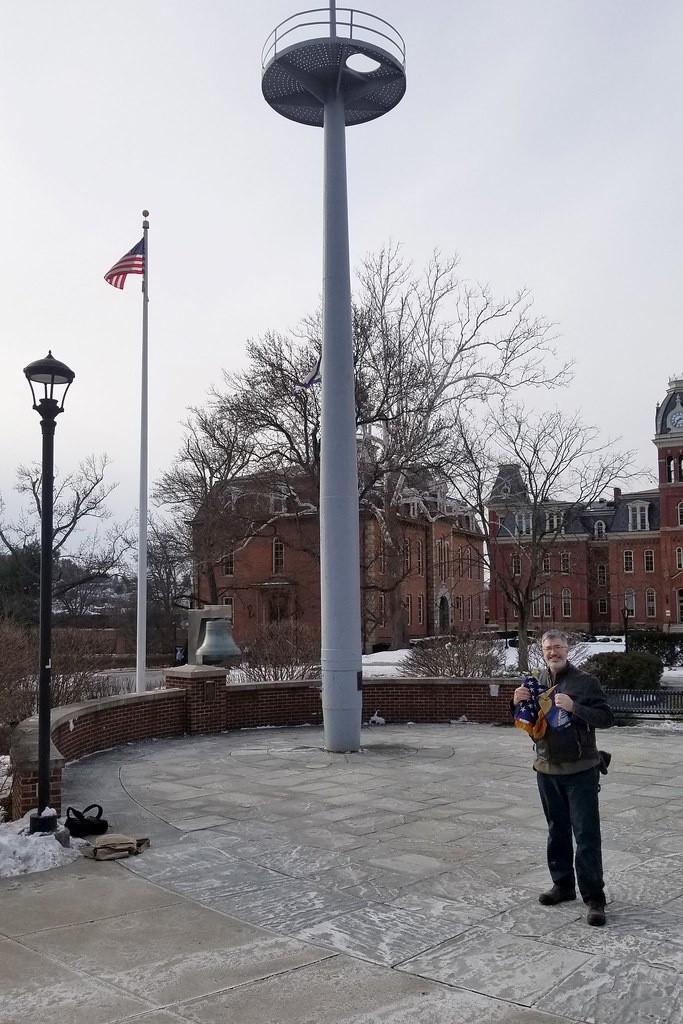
[104,238,145,290]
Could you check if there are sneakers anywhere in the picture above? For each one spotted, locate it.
[539,883,576,905]
[587,905,607,925]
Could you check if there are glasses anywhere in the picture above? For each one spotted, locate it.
[542,646,566,652]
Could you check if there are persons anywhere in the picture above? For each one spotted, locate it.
[509,629,617,926]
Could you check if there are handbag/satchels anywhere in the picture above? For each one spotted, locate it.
[84,833,147,861]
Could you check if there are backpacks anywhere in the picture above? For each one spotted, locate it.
[64,804,108,838]
